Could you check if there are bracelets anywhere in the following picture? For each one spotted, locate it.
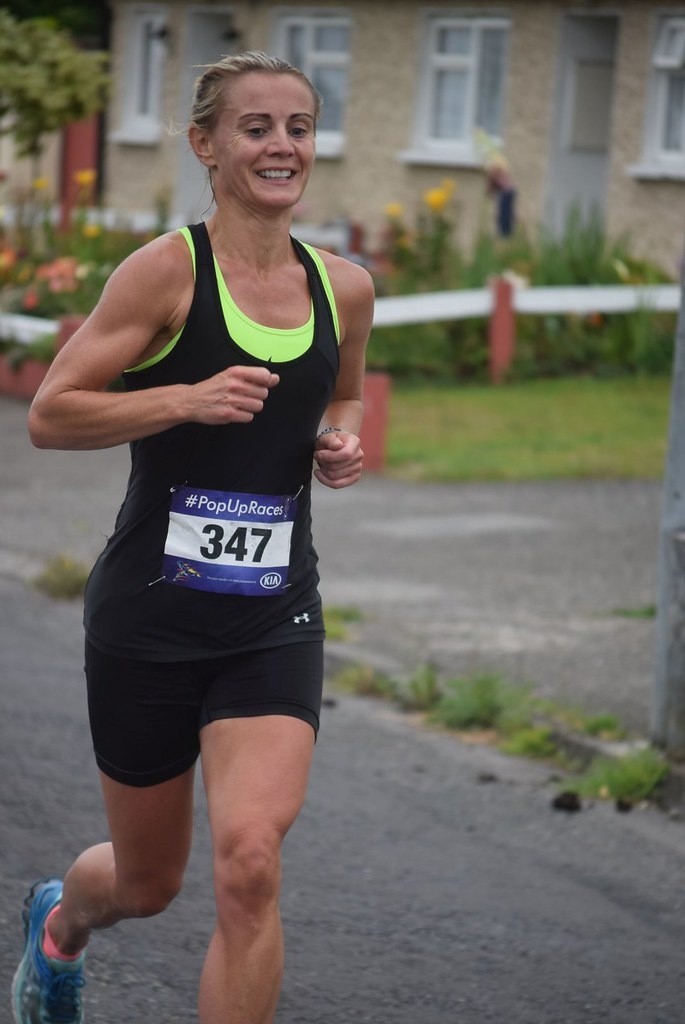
[316,427,344,440]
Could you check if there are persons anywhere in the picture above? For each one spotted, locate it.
[10,49,376,1024]
[481,157,517,238]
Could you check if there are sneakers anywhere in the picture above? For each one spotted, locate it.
[9,877,88,1024]
